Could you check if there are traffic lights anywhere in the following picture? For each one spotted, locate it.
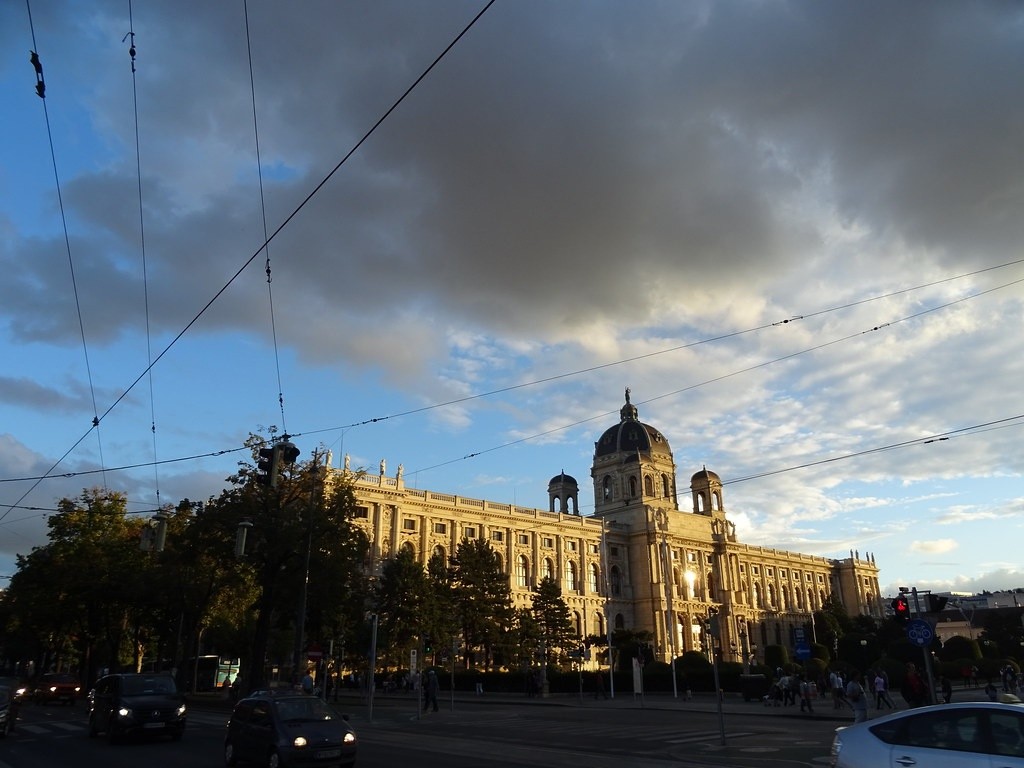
[704,614,719,638]
[256,447,278,491]
[924,594,948,612]
[284,447,300,462]
[425,639,433,653]
[892,596,911,627]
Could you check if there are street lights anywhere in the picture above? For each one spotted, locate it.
[731,620,757,675]
[860,640,869,669]
[984,640,990,658]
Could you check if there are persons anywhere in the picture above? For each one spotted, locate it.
[528,670,545,698]
[901,661,952,709]
[999,665,1018,696]
[303,669,314,694]
[773,666,828,713]
[327,450,333,465]
[625,385,631,404]
[830,669,898,709]
[475,672,483,695]
[846,671,870,723]
[598,670,610,699]
[345,453,350,469]
[232,673,240,699]
[397,463,404,479]
[960,664,981,688]
[223,676,231,697]
[319,668,411,696]
[380,460,386,475]
[423,671,440,712]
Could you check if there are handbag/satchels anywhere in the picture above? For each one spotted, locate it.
[943,692,950,699]
[1006,673,1012,681]
[920,680,931,704]
[972,671,976,676]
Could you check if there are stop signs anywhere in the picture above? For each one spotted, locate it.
[307,644,323,662]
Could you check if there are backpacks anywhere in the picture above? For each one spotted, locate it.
[987,686,997,697]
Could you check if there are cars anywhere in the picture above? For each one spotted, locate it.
[27,673,82,705]
[0,677,26,704]
[0,686,20,737]
[224,691,358,768]
[829,693,1024,768]
[87,673,187,745]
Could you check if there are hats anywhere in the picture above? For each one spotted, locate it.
[849,669,860,679]
[429,671,434,674]
